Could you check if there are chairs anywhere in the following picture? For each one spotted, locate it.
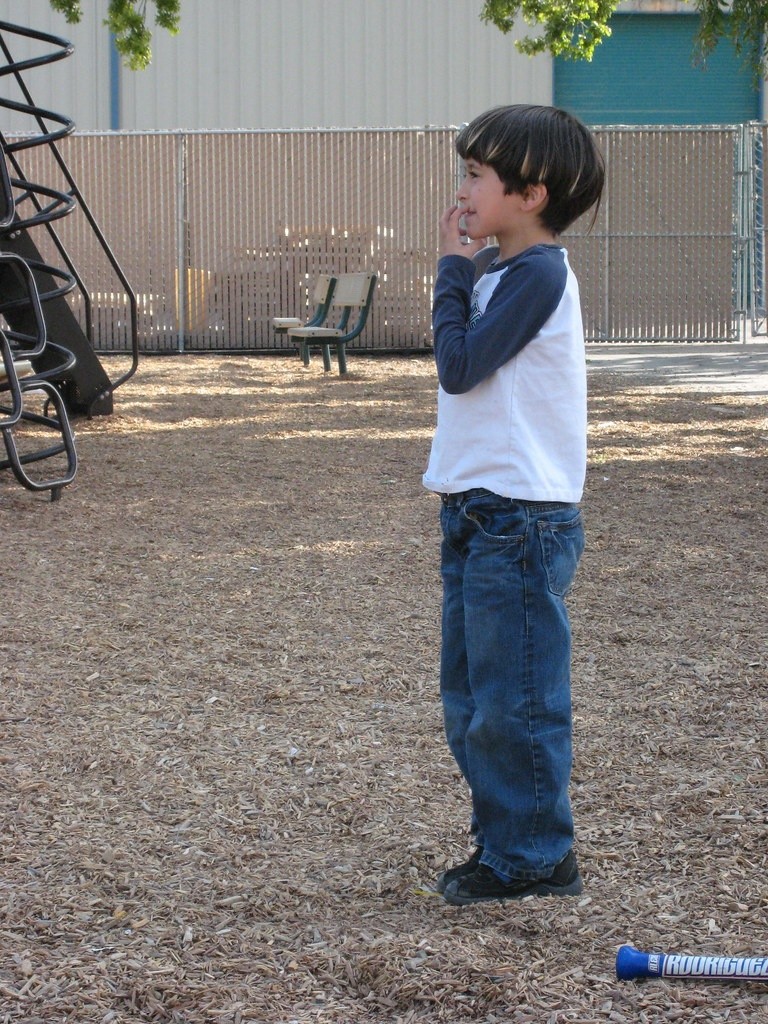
[287,272,377,374]
[273,274,338,365]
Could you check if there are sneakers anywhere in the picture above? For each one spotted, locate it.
[433,843,482,893]
[440,849,586,905]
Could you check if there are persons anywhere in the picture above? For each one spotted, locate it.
[422,104,606,906]
